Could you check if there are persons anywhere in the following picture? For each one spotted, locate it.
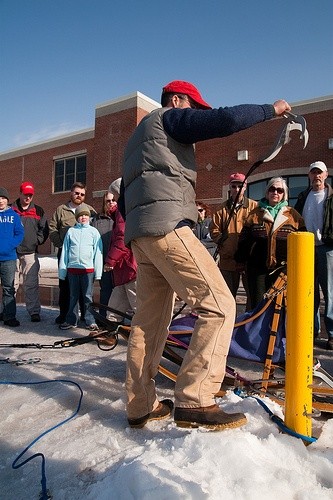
[103,177,137,324]
[209,174,259,313]
[57,205,103,330]
[91,191,117,319]
[194,200,217,255]
[0,187,25,326]
[235,177,308,312]
[294,161,333,349]
[49,183,99,323]
[117,81,291,429]
[8,182,49,322]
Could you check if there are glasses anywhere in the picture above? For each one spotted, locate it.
[75,192,85,196]
[107,199,115,202]
[198,209,204,213]
[179,96,198,109]
[231,184,244,189]
[269,186,285,194]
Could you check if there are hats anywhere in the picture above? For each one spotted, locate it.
[162,80,213,110]
[308,160,328,172]
[20,181,35,195]
[75,204,90,219]
[228,172,247,185]
[108,178,122,196]
[0,186,10,203]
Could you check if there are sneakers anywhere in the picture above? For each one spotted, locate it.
[127,399,174,428]
[174,404,248,431]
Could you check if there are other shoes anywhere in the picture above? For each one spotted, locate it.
[325,338,333,350]
[81,323,99,331]
[58,322,78,330]
[30,314,41,322]
[4,318,20,327]
[89,329,116,345]
[55,315,65,323]
